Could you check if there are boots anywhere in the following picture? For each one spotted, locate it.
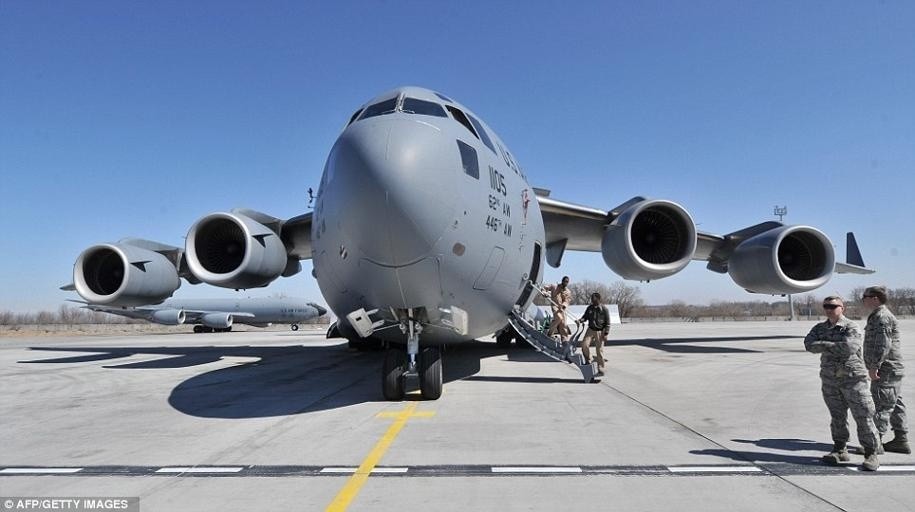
[861,446,882,471]
[822,440,851,462]
[881,431,912,454]
[855,433,885,454]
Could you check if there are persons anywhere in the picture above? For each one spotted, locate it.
[860,285,912,455]
[575,292,610,377]
[803,296,885,471]
[540,275,572,346]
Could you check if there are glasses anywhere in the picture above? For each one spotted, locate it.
[822,302,844,310]
[863,294,874,298]
